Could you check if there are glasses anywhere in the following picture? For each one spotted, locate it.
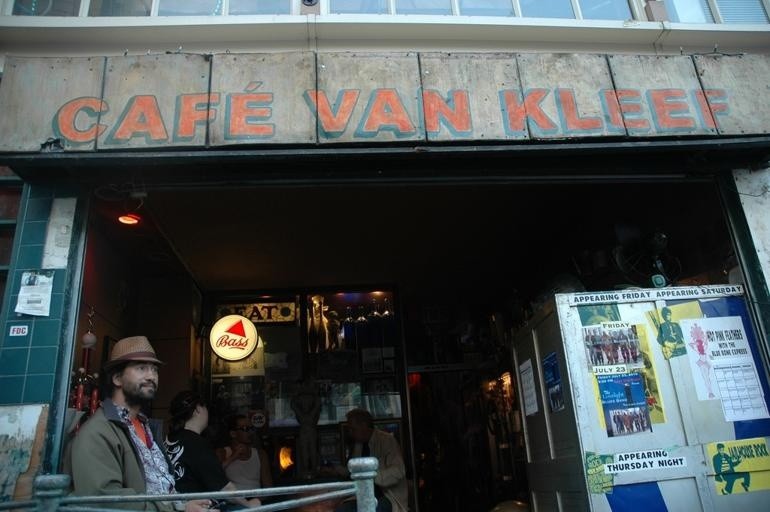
[234,425,256,432]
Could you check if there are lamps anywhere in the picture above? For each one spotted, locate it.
[117,191,146,226]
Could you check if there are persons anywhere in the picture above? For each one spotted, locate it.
[322,409,409,511]
[214,414,274,505]
[166,390,257,511]
[71,335,213,511]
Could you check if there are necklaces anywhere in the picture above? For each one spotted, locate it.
[240,447,250,460]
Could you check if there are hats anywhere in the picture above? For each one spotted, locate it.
[99,336,172,375]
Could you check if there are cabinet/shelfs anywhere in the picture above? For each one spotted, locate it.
[201,282,418,512]
